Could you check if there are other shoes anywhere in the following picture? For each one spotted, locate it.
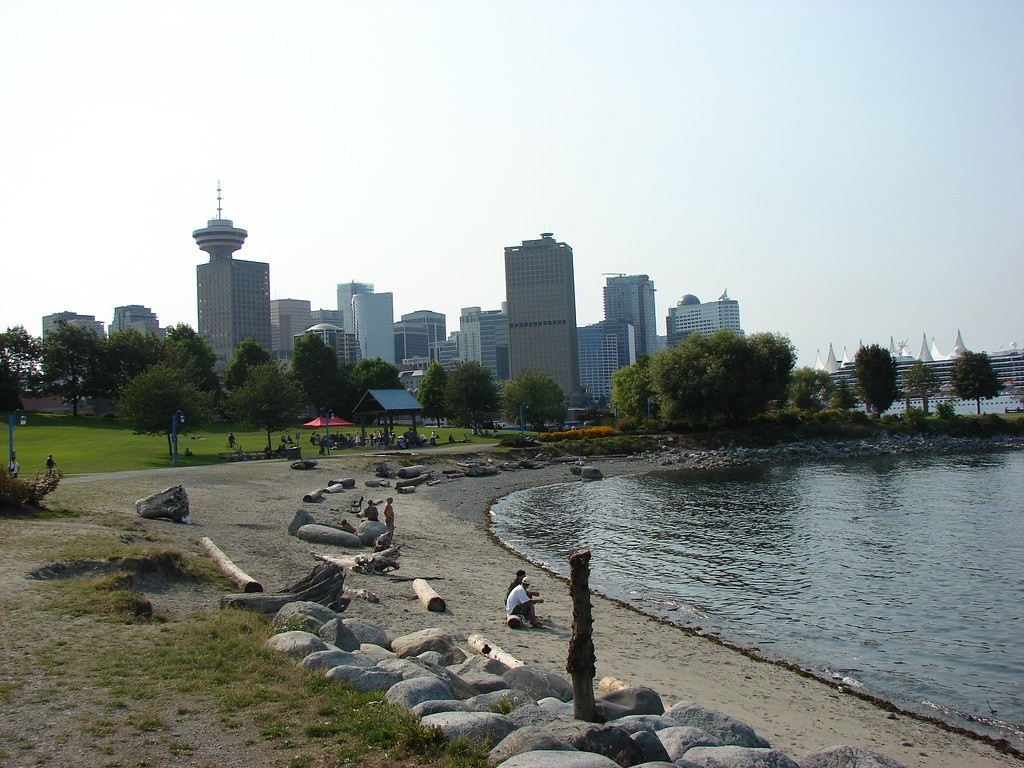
[534,623,544,629]
[529,621,542,625]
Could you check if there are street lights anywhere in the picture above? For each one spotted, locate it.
[325,408,334,454]
[172,410,185,453]
[8,408,27,463]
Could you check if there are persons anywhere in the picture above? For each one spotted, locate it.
[356,429,395,446]
[310,431,354,456]
[184,448,192,456]
[341,519,356,533]
[398,427,436,449]
[45,454,57,473]
[384,497,394,536]
[7,457,20,478]
[505,569,548,629]
[237,446,245,461]
[449,433,468,443]
[472,426,499,437]
[228,432,236,448]
[281,434,293,444]
[191,435,201,439]
[264,444,286,459]
[364,500,380,522]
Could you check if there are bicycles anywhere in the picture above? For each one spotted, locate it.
[226,440,239,450]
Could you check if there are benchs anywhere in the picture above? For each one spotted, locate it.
[218,449,277,456]
[365,438,383,446]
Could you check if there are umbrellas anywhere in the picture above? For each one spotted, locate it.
[303,416,326,436]
[320,415,354,434]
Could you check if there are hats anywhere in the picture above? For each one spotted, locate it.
[522,576,530,585]
